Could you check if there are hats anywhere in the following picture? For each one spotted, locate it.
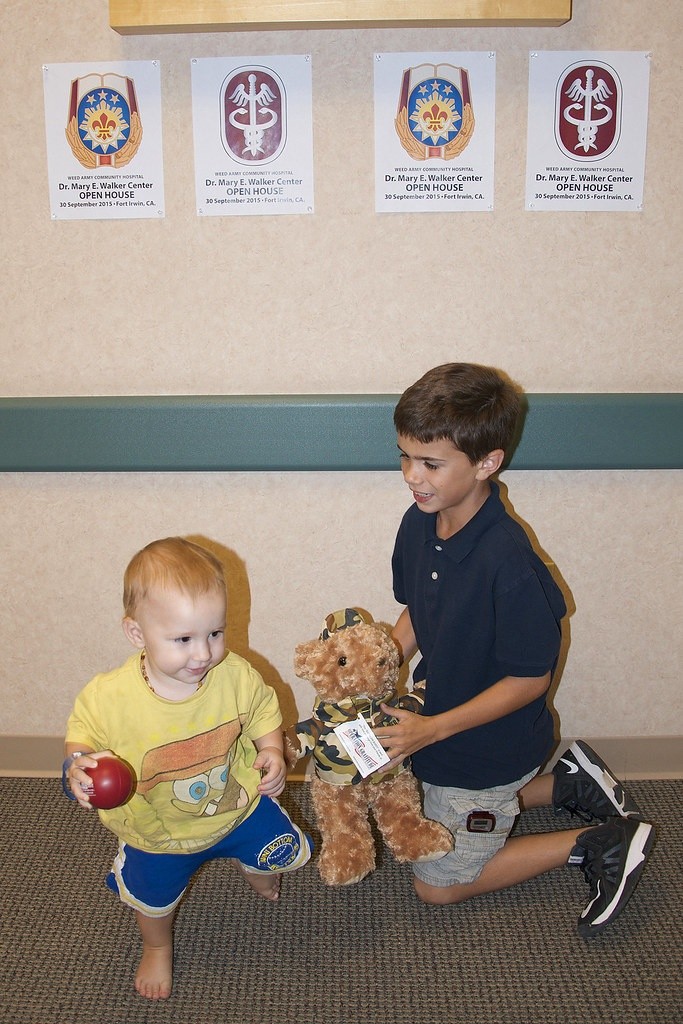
[319,608,364,640]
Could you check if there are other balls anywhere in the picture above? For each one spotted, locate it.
[80,757,134,810]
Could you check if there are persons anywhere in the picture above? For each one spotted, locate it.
[370,361,658,939]
[61,538,314,1002]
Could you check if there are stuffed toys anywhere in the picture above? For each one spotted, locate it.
[282,608,456,887]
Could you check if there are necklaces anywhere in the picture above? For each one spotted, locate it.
[141,653,202,696]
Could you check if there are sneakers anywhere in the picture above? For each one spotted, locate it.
[552,740,642,822]
[565,818,656,934]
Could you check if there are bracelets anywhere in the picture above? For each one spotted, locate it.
[62,751,86,800]
